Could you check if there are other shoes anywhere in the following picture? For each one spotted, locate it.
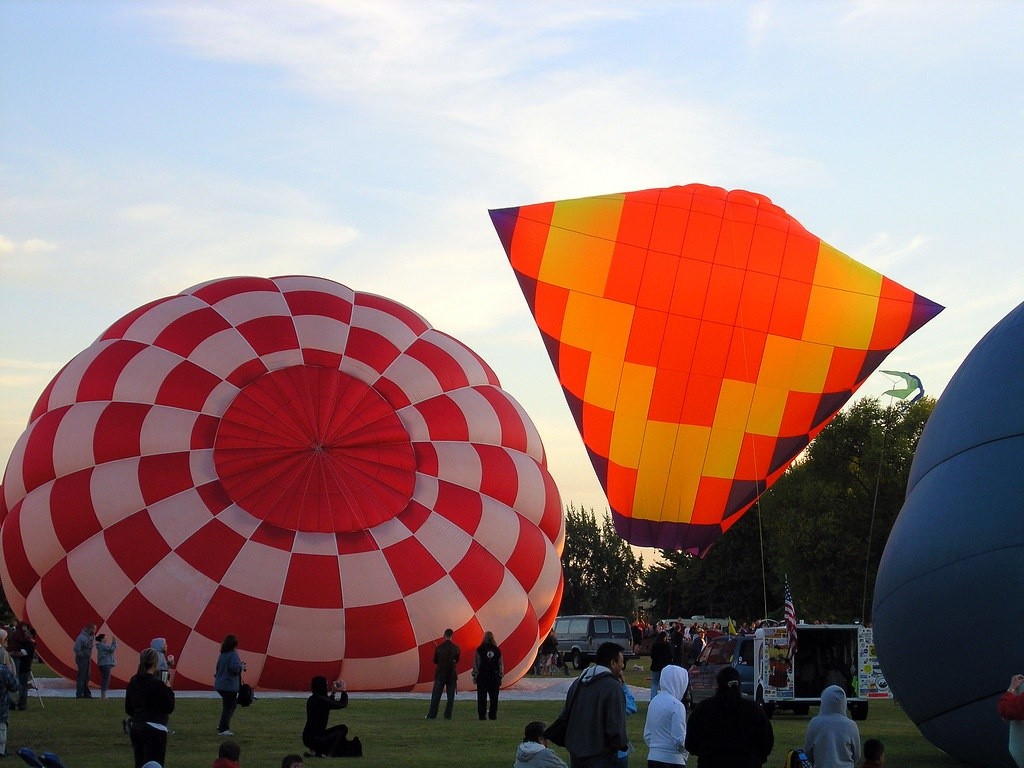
[219,730,234,737]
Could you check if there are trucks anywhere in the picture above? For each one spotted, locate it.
[749,621,894,721]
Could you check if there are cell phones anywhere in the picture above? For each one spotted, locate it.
[335,682,341,689]
[160,670,170,683]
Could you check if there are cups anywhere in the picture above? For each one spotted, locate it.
[167,655,174,665]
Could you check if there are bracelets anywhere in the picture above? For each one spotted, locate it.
[1008,688,1016,694]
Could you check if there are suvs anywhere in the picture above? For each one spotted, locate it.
[549,613,637,671]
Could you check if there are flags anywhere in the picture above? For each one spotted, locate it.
[785,582,798,653]
[729,620,735,634]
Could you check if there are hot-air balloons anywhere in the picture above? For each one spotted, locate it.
[488,182,947,663]
[871,298,1024,768]
[0,273,567,694]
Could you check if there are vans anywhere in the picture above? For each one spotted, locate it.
[683,633,755,710]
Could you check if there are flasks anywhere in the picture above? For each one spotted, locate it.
[159,669,168,684]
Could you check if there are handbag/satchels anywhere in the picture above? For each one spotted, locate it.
[239,683,255,707]
[540,670,587,747]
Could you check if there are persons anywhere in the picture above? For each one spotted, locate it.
[0,621,37,757]
[74,625,96,699]
[862,739,885,768]
[151,638,174,671]
[999,675,1024,768]
[212,741,240,768]
[214,635,248,736]
[302,676,348,758]
[685,667,774,768]
[805,685,860,768]
[514,721,568,768]
[425,629,460,719]
[644,665,689,768]
[125,649,175,768]
[531,627,570,676]
[559,642,637,768]
[282,755,304,768]
[95,634,117,700]
[632,617,778,714]
[471,632,503,720]
